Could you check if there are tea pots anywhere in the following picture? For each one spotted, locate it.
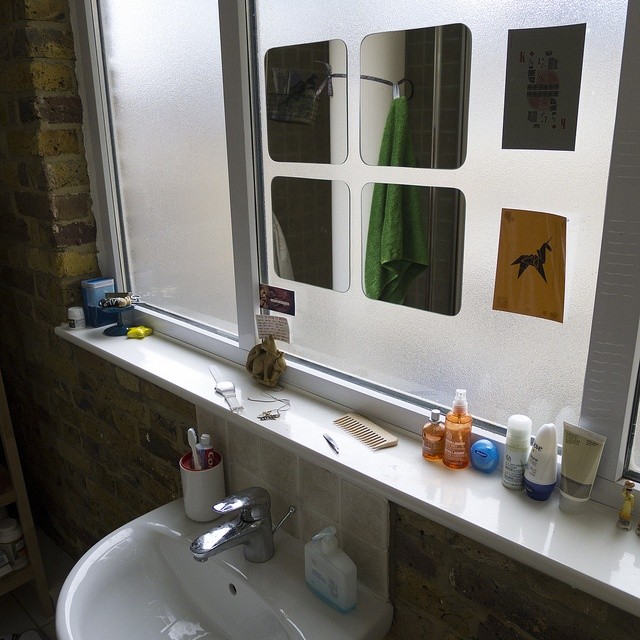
[272,174,350,293]
[360,23,473,169]
[360,182,466,316]
[265,40,350,165]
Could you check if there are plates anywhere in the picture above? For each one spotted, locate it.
[0,372,57,619]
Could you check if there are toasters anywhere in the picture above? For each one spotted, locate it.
[186,427,203,471]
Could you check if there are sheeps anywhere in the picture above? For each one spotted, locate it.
[190,485,274,563]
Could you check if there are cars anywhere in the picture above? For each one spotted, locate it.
[178,450,226,523]
